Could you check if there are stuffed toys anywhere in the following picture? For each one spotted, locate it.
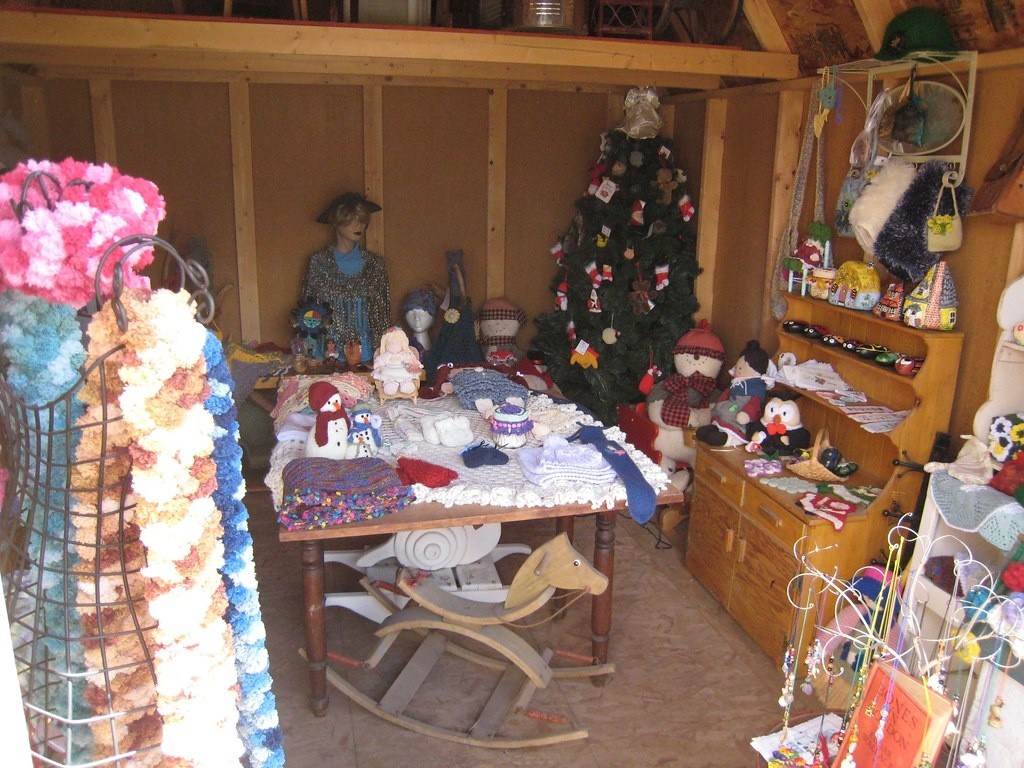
[473,296,528,368]
[347,429,370,459]
[304,381,350,461]
[746,389,812,457]
[782,219,830,273]
[347,407,381,458]
[645,319,777,446]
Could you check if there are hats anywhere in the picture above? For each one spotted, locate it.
[402,287,439,317]
[315,192,383,224]
[491,403,535,437]
[872,6,959,63]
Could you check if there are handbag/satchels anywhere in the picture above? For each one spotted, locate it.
[927,182,962,252]
[770,229,834,323]
[966,149,1024,225]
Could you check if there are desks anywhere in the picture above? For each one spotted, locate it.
[277,372,685,716]
[756,707,959,768]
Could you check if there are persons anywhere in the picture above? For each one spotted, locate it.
[402,289,439,354]
[298,191,393,364]
[371,324,424,395]
[843,564,902,674]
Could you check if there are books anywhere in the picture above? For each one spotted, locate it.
[830,663,955,768]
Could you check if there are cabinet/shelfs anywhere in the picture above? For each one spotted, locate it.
[897,470,1023,768]
[685,291,964,681]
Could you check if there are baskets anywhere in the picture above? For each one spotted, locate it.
[786,424,849,483]
